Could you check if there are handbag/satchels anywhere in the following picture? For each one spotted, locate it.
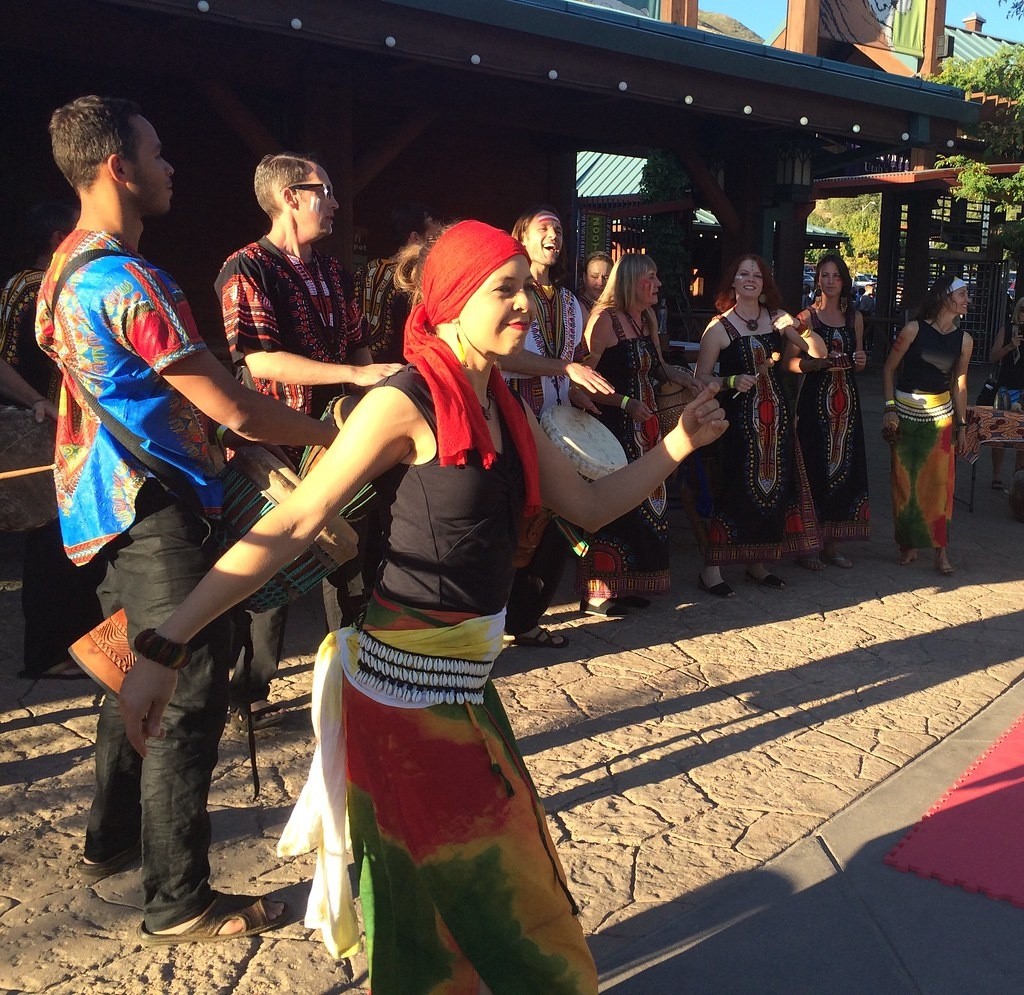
[652,364,694,411]
[975,380,999,406]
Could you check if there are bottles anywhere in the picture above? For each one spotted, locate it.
[656,298,667,335]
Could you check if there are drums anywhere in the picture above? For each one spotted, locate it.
[0,403,60,534]
[509,403,632,569]
[656,363,694,438]
[64,444,360,702]
[293,392,402,524]
[1006,463,1024,522]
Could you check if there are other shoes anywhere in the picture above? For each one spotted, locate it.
[579,597,629,617]
[992,480,1005,489]
[1015,463,1024,472]
[637,597,652,608]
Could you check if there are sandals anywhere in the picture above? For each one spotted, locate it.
[231,700,291,731]
[516,626,570,648]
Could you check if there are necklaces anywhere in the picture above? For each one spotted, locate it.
[936,322,954,334]
[733,304,761,331]
[623,311,649,346]
[481,386,495,420]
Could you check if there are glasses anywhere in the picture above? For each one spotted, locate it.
[291,182,333,200]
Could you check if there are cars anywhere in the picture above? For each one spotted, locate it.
[803,261,1024,315]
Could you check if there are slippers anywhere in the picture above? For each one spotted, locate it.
[17,659,90,680]
[698,573,737,599]
[77,836,142,878]
[138,889,290,947]
[820,552,854,569]
[747,573,786,589]
[795,554,826,570]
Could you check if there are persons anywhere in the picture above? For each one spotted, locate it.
[492,207,616,647]
[212,157,449,733]
[1,99,342,947]
[803,284,820,307]
[118,219,729,995]
[686,254,823,597]
[783,255,871,570]
[882,277,973,575]
[991,298,1024,489]
[852,287,876,312]
[576,251,614,330]
[575,253,707,615]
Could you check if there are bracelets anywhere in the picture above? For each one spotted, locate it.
[795,322,811,337]
[135,628,193,670]
[620,396,630,409]
[722,375,736,389]
[957,422,967,427]
[884,400,897,413]
